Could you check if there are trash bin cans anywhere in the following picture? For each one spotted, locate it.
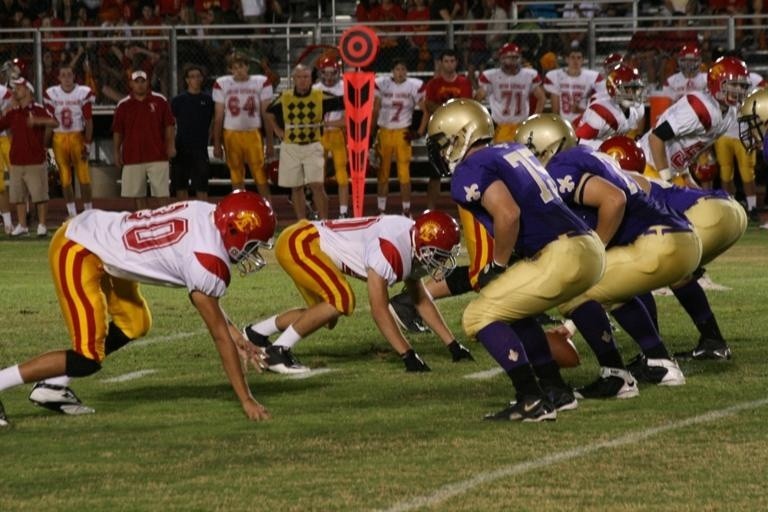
[91,164,119,200]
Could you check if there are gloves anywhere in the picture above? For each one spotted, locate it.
[400,348,433,373]
[446,337,474,364]
[475,256,509,292]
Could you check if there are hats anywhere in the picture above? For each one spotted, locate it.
[127,67,148,81]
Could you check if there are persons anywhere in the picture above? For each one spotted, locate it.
[1,187,280,429]
[512,111,705,400]
[388,203,496,335]
[620,167,749,361]
[240,209,476,376]
[425,95,608,424]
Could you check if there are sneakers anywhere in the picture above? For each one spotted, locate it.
[27,379,97,416]
[10,224,30,236]
[674,339,734,362]
[388,295,433,338]
[628,352,688,389]
[544,383,578,411]
[257,344,312,375]
[37,222,48,237]
[571,365,642,400]
[485,393,558,424]
[240,323,272,349]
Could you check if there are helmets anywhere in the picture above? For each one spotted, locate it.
[318,55,340,87]
[413,206,462,283]
[3,58,26,80]
[514,111,579,168]
[736,86,768,152]
[596,134,647,175]
[707,55,752,109]
[497,44,523,78]
[213,188,278,277]
[603,62,645,106]
[424,97,496,179]
[692,160,722,184]
[603,52,623,72]
[678,43,704,80]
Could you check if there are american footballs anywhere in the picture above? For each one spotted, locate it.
[543,331,579,367]
